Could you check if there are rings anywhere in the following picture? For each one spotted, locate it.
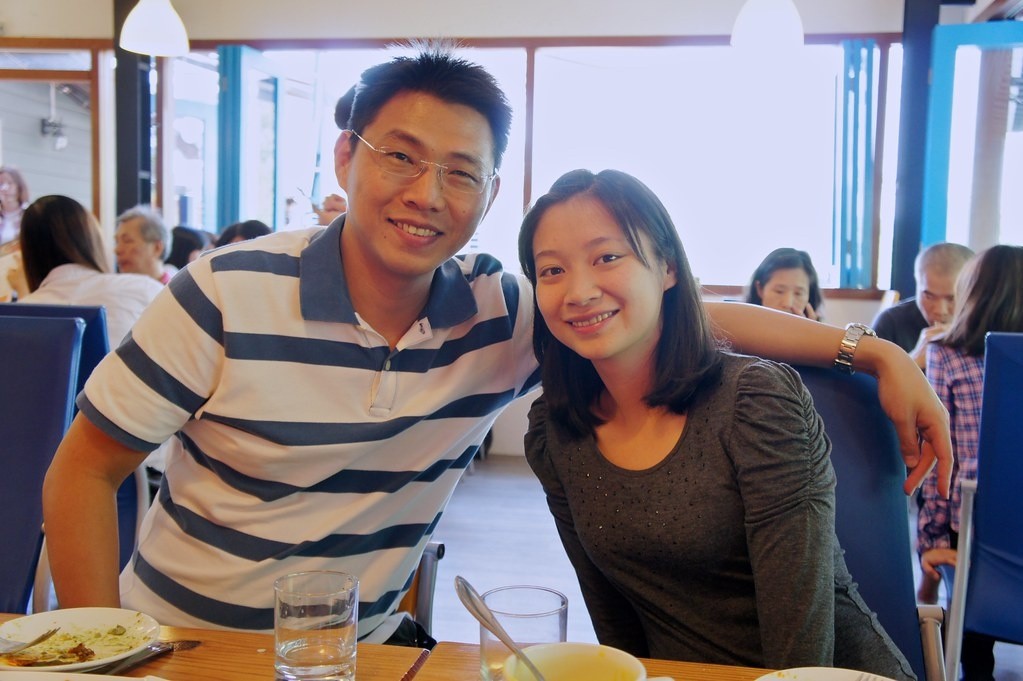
[816,316,823,321]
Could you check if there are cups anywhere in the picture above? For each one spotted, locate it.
[273,570,362,681]
[503,641,675,681]
[478,584,569,680]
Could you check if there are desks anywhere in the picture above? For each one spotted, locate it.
[0,615,882,681]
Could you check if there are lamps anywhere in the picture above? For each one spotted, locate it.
[729,1,803,50]
[118,0,190,58]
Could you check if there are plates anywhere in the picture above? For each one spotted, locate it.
[0,607,162,673]
[754,666,897,681]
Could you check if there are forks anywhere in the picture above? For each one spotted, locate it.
[0,627,62,655]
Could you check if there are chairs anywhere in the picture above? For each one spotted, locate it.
[795,367,947,676]
[943,328,1023,681]
[0,302,150,614]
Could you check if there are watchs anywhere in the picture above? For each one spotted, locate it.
[835,322,876,375]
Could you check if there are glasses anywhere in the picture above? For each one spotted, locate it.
[351,129,496,195]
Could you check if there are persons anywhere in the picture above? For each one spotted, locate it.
[872,243,975,377]
[42,54,953,645]
[917,245,1023,605]
[517,169,916,681]
[747,247,825,322]
[165,220,272,273]
[0,166,30,245]
[113,208,172,285]
[20,197,170,505]
